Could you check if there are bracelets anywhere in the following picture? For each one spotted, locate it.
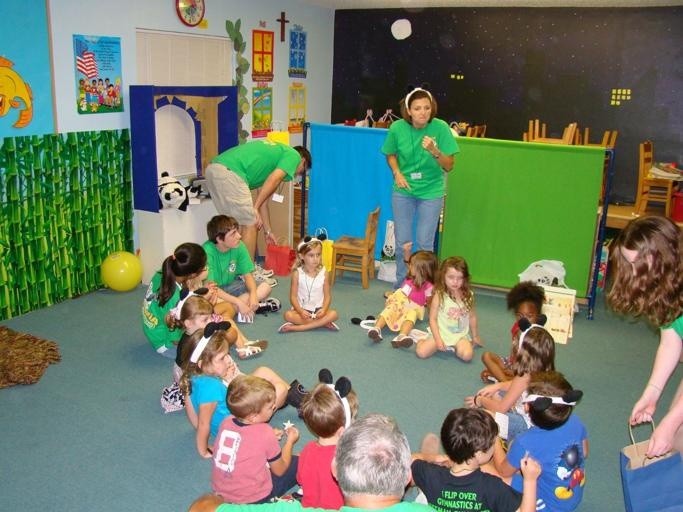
[646,384,663,392]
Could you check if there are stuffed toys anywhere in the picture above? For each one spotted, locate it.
[157,171,189,211]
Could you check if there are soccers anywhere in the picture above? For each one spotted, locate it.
[100,252,141,293]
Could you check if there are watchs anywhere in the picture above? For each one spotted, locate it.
[433,151,441,159]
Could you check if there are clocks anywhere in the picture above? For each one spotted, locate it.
[176,0,205,27]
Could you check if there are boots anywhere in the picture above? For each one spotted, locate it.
[288,379,310,409]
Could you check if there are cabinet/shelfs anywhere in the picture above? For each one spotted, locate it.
[251,179,309,255]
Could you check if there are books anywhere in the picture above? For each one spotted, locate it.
[647,163,683,179]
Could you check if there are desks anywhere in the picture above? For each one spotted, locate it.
[587,204,683,295]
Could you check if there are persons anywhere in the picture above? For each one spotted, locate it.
[180,321,542,512]
[382,89,460,298]
[464,281,589,510]
[279,234,340,333]
[368,250,484,361]
[204,139,312,288]
[143,215,282,414]
[607,215,683,459]
[78,78,120,112]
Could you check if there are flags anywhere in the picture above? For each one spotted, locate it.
[76,39,98,78]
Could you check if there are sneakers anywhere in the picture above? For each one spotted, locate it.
[277,322,294,333]
[407,328,428,344]
[239,261,281,314]
[328,322,340,331]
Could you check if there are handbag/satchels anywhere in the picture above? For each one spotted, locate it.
[264,243,296,275]
[620,418,683,512]
[315,227,334,272]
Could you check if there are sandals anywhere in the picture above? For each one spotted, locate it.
[245,339,268,348]
[367,327,383,343]
[236,346,264,360]
[391,334,413,349]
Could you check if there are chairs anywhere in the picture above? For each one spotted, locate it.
[331,208,379,289]
[634,139,673,217]
[522,118,618,202]
